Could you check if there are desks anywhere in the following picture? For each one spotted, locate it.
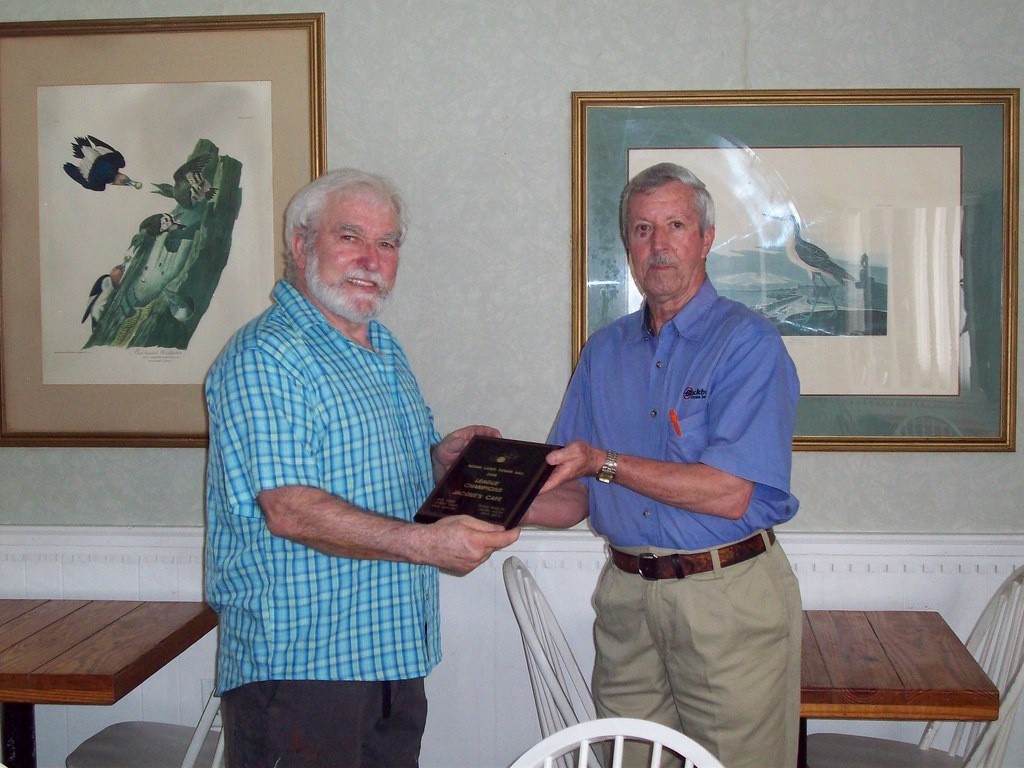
[797,610,1000,768]
[0,598,220,768]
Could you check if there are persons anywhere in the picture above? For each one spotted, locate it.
[205,168,521,768]
[519,163,803,768]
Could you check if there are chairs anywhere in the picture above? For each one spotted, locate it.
[501,556,727,768]
[805,563,1024,768]
[65,677,229,768]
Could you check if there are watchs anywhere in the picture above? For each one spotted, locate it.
[596,450,618,484]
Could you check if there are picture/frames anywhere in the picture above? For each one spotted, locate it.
[0,10,329,449]
[568,87,1018,459]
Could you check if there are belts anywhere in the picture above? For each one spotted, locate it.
[609,527,776,580]
